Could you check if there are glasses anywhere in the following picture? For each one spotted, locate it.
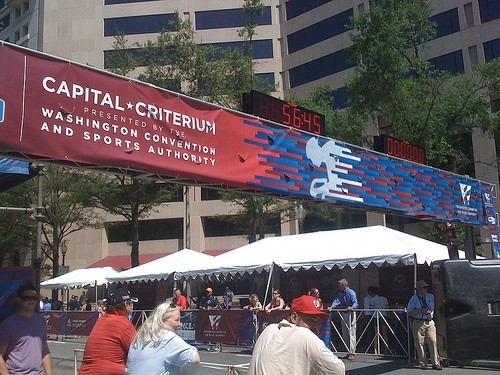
[21,296,38,301]
[168,303,176,309]
[273,293,278,294]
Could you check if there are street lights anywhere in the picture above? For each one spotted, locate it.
[59,240,68,305]
[474,240,495,259]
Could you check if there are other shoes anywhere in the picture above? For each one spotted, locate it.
[348,354,355,360]
[421,365,428,369]
[432,365,442,370]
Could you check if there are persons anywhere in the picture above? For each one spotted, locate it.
[172,289,187,310]
[265,289,286,315]
[247,296,345,375]
[68,295,91,311]
[127,303,200,375]
[199,288,219,351]
[363,286,375,353]
[222,286,233,309]
[0,285,52,375]
[408,280,443,369]
[98,304,105,317]
[243,294,262,312]
[369,287,390,355]
[328,278,359,360]
[40,296,63,310]
[307,288,323,311]
[78,288,138,375]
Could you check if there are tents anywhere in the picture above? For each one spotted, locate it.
[105,248,216,298]
[40,266,118,309]
[174,224,486,307]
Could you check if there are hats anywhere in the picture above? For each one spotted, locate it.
[205,288,212,292]
[413,280,429,290]
[109,288,138,304]
[290,295,330,318]
